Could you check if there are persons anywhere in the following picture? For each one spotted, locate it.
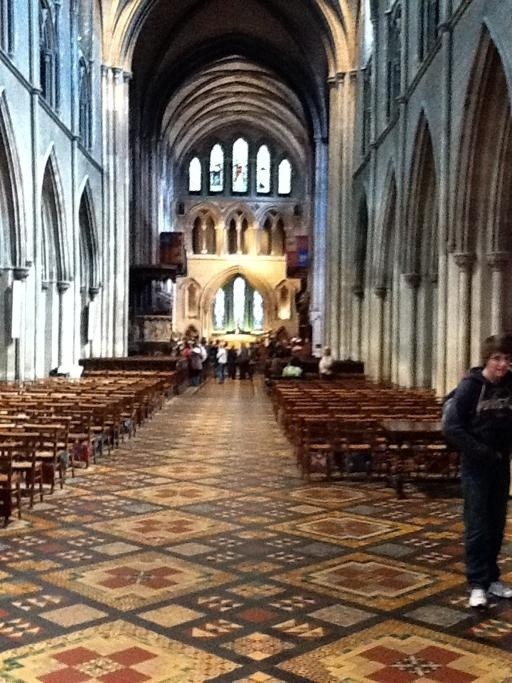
[174,336,257,385]
[442,333,512,607]
[318,346,336,379]
[281,357,303,377]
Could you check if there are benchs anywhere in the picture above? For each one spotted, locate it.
[1,366,178,528]
[265,356,466,498]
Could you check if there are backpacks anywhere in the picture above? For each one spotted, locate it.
[440,383,483,453]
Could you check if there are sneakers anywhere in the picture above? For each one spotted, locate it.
[469,588,488,608]
[488,580,512,598]
